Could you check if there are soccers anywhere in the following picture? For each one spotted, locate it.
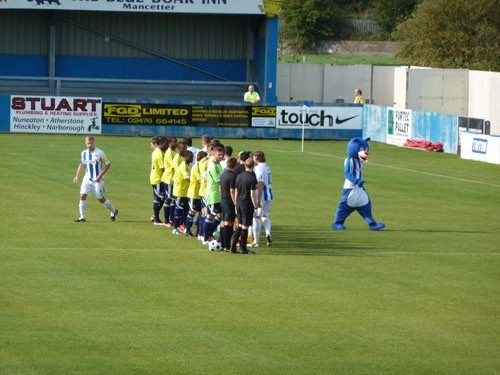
[208,240,221,251]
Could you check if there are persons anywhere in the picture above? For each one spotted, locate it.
[353,88,365,104]
[73,136,121,223]
[148,133,273,255]
[244,85,262,103]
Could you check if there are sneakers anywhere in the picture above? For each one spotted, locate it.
[150,215,274,256]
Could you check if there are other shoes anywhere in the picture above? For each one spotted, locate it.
[110,209,118,221]
[74,219,85,222]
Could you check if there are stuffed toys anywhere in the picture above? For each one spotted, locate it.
[331,138,387,231]
